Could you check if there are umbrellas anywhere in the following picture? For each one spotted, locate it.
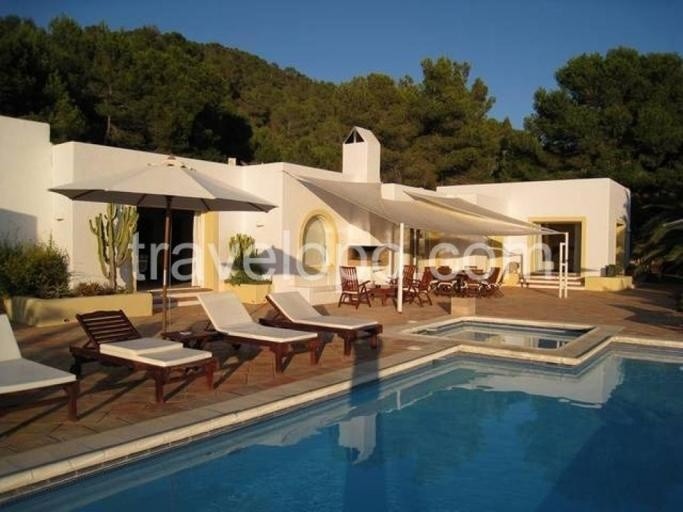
[50,154,282,339]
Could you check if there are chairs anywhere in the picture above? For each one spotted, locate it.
[337,265,507,310]
